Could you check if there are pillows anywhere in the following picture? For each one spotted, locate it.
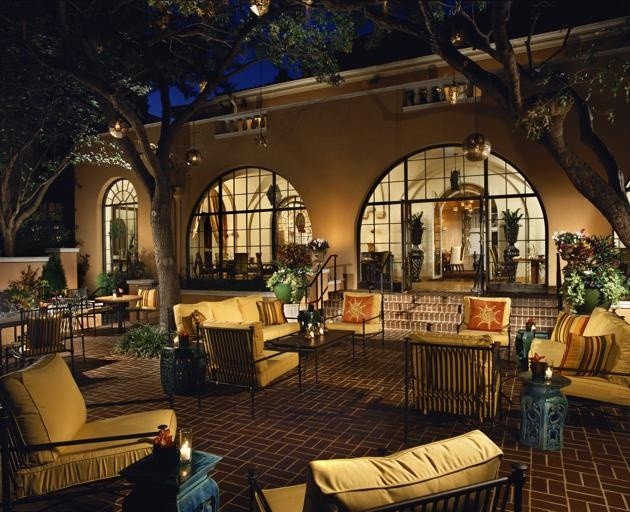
[528,308,630,387]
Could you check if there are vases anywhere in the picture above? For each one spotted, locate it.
[574,287,612,315]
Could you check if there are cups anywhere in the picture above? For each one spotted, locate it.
[112,290,117,298]
[178,427,193,463]
[544,360,554,381]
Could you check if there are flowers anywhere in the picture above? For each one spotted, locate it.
[553,227,629,315]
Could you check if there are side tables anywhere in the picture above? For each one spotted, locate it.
[517,370,571,451]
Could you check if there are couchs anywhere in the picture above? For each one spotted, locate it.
[517,308,630,431]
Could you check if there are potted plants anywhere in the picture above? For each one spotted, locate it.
[500,208,524,283]
[406,211,426,282]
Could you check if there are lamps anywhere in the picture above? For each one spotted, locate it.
[452,200,473,212]
[106,0,271,168]
[443,34,492,161]
